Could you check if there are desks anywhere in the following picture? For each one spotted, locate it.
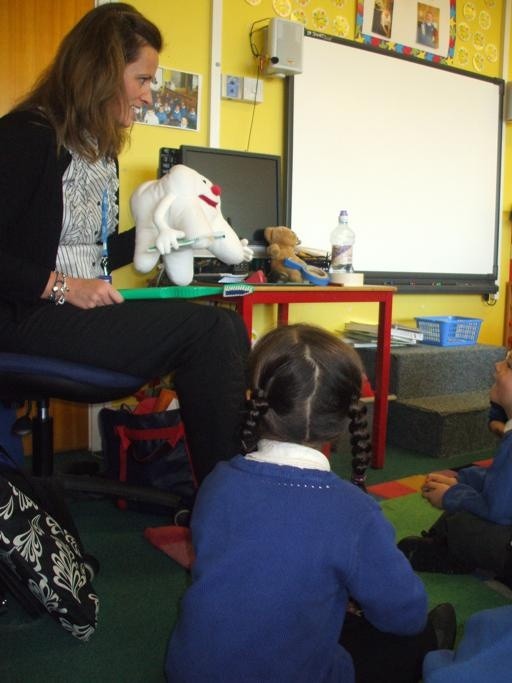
[155,281,398,471]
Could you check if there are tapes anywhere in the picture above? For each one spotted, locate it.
[284,257,329,285]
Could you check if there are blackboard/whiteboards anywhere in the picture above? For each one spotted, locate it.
[282,27,504,292]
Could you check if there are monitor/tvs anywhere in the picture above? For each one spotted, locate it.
[179,145,283,258]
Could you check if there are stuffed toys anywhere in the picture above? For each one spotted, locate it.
[263,225,307,282]
[130,164,254,287]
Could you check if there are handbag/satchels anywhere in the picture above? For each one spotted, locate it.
[99,403,197,512]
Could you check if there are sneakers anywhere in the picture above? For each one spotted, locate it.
[429,602,456,650]
[397,529,446,574]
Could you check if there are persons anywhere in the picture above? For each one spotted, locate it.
[162,322,461,682]
[136,91,196,128]
[0,0,253,488]
[417,8,437,48]
[397,348,512,588]
[378,5,391,38]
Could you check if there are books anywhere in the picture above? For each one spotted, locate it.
[334,319,424,351]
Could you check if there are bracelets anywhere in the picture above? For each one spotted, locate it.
[54,272,71,307]
[49,270,60,302]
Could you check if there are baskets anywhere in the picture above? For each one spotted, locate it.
[413,315,483,347]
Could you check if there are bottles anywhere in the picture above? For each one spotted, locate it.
[328,210,355,276]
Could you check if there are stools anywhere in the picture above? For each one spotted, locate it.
[0,351,193,528]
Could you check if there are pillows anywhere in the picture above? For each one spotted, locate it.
[0,477,102,642]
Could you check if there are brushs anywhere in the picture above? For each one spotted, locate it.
[118,283,253,299]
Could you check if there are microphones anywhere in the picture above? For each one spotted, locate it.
[257,53,279,64]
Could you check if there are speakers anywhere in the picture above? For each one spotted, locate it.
[507,80,512,121]
[264,17,304,75]
